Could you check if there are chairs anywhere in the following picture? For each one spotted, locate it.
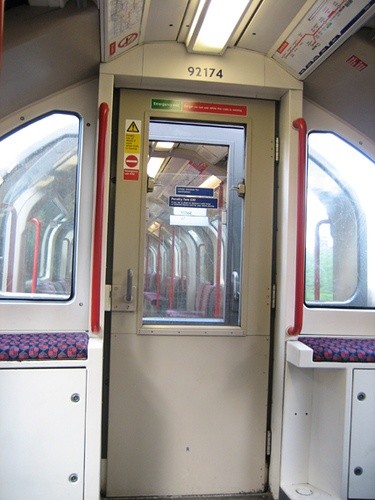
[22,272,221,321]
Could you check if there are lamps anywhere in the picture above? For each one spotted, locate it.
[144,140,222,191]
[183,0,253,57]
